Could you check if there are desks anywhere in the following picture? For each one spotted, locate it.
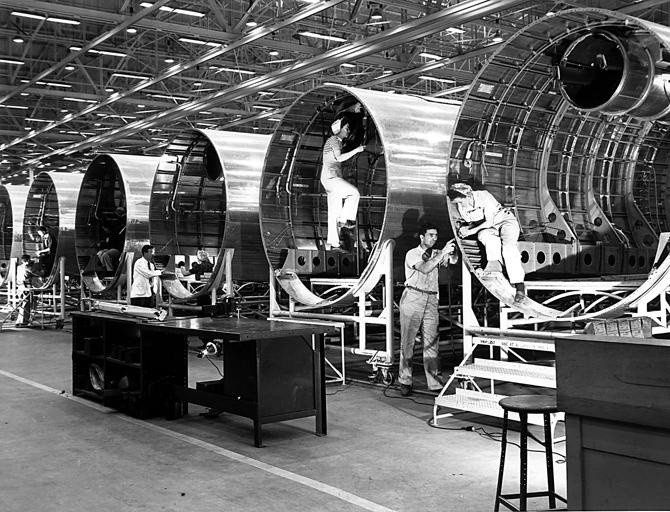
[71,309,335,447]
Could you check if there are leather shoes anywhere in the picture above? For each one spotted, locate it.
[329,246,351,254]
[513,288,527,307]
[336,220,356,229]
[400,382,413,396]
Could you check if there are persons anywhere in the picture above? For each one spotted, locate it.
[15,255,39,326]
[27,226,54,274]
[178,262,191,286]
[447,182,526,302]
[0,263,8,276]
[129,243,164,308]
[398,226,460,397]
[190,261,201,274]
[320,116,366,249]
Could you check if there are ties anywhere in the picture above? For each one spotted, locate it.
[147,261,153,285]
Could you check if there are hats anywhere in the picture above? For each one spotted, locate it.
[196,250,210,261]
[331,117,345,135]
[449,182,474,198]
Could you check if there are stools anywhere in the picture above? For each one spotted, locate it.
[494,394,569,512]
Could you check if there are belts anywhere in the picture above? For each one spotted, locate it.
[405,285,439,295]
